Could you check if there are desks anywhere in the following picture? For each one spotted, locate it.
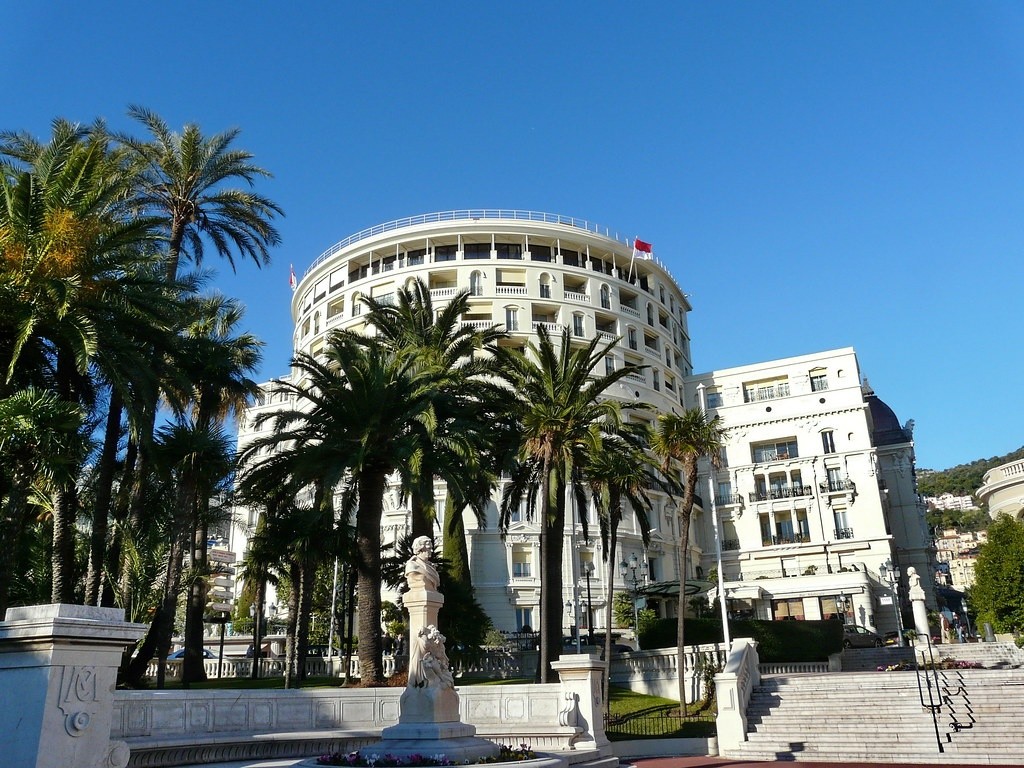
[775,454,782,461]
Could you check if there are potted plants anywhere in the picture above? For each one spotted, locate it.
[904,629,919,647]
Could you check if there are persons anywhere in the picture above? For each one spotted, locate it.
[939,611,969,644]
[404,535,455,689]
[907,566,920,588]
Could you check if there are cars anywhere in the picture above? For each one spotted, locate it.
[166,647,229,660]
[277,643,344,671]
[841,623,886,650]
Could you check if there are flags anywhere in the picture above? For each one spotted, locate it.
[288,268,298,291]
[634,238,652,254]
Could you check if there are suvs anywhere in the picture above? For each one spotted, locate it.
[884,629,913,648]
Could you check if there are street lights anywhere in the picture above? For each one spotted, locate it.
[878,557,909,647]
[583,560,595,646]
[961,598,972,638]
[620,552,649,643]
